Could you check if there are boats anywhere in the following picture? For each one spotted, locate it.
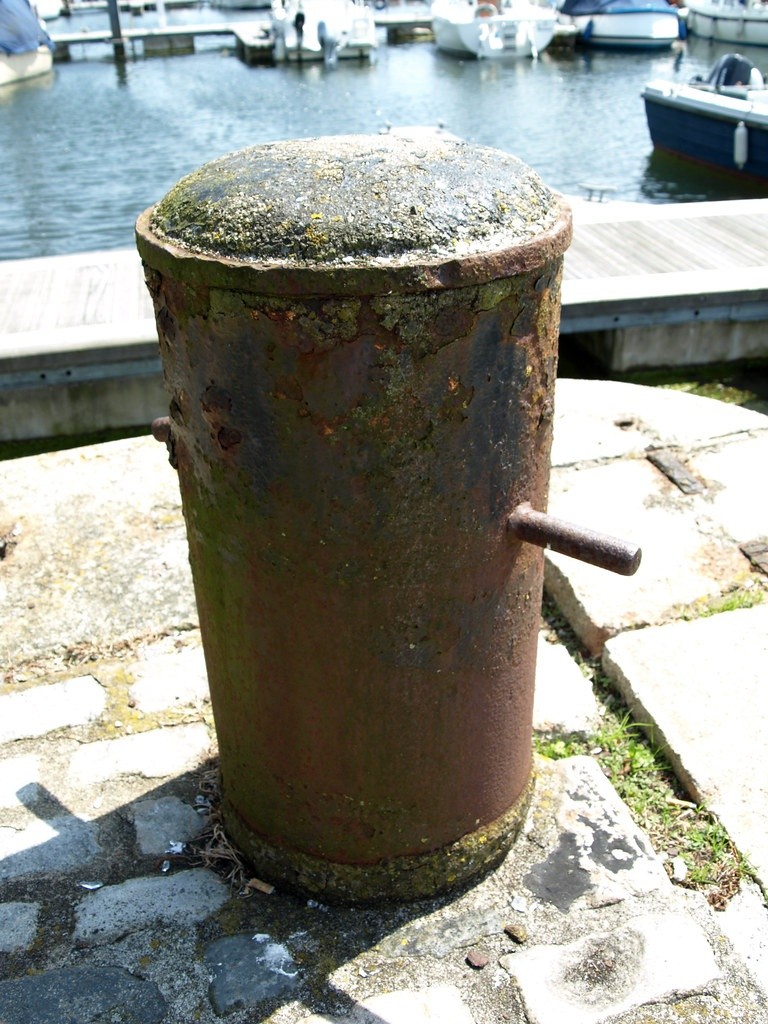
[641,53,768,185]
[430,0,558,60]
[538,0,679,49]
[683,0,768,46]
